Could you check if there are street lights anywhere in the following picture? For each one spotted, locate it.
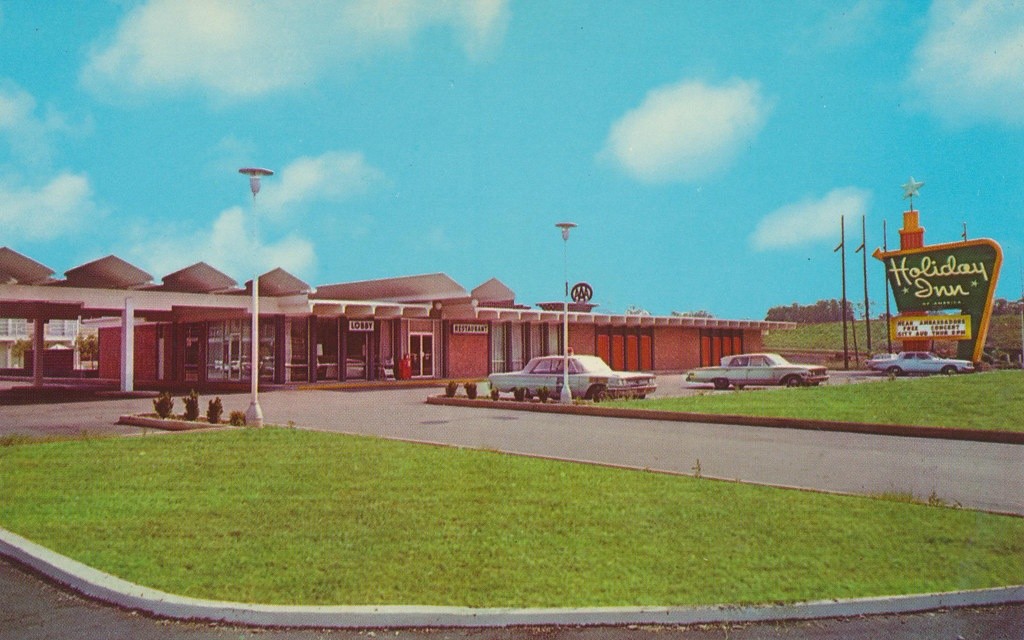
[238,168,275,428]
[554,221,578,403]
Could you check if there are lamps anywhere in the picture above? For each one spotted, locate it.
[472,298,478,306]
[435,301,443,310]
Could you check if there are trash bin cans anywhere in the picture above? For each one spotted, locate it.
[397,353,411,379]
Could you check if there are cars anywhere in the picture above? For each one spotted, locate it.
[685,352,831,389]
[863,350,976,376]
[488,354,659,403]
